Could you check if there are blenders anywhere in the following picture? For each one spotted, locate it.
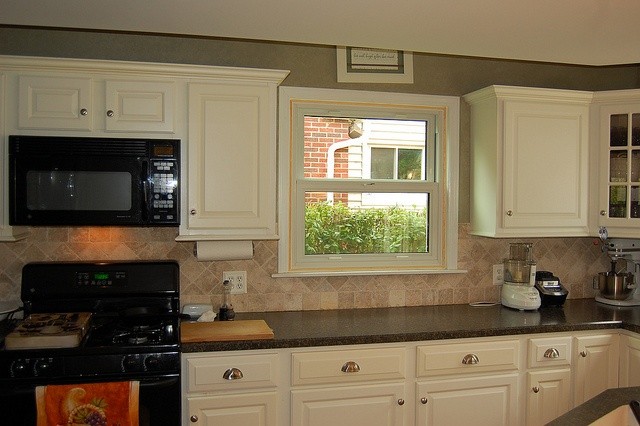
[501,242,541,311]
[593,238,640,307]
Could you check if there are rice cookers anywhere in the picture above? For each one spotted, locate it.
[534,270,568,306]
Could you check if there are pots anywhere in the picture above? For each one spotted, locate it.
[592,271,636,300]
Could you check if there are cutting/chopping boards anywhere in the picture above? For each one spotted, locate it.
[179,319,274,343]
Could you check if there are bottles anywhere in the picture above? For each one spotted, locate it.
[609,158,627,218]
[631,157,640,218]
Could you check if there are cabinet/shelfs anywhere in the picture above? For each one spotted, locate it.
[573,333,620,409]
[17,74,176,134]
[597,91,640,239]
[414,339,519,425]
[290,346,408,426]
[502,97,591,229]
[186,352,279,425]
[525,335,572,426]
[186,81,276,240]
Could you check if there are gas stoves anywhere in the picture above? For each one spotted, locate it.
[0,299,181,353]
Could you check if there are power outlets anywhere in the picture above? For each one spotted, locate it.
[223,270,248,295]
[493,264,504,286]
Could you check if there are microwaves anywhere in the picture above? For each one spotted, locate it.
[8,135,181,227]
